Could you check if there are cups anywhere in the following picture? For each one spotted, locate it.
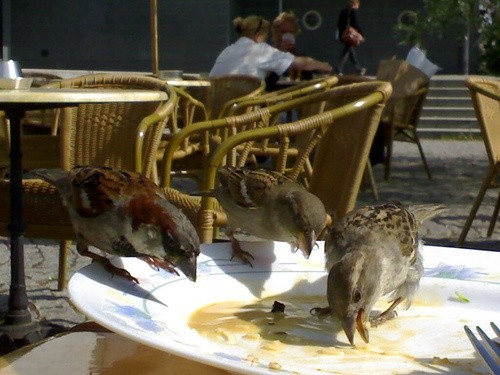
[289,68,302,82]
[0,59,22,78]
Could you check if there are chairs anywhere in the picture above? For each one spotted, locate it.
[0,61,500,291]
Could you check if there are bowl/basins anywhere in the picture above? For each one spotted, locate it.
[0,77,33,90]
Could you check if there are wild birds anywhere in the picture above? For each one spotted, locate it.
[307,199,451,347]
[29,161,202,285]
[187,162,331,270]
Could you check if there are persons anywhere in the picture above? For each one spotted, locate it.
[334,0,367,78]
[203,15,333,149]
[261,11,319,103]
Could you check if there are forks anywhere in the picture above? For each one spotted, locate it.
[462,320,500,375]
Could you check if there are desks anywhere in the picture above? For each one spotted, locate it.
[0,91,168,325]
[0,319,241,375]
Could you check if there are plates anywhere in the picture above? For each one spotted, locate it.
[66,239,500,375]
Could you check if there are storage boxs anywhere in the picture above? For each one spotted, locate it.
[377,59,429,125]
